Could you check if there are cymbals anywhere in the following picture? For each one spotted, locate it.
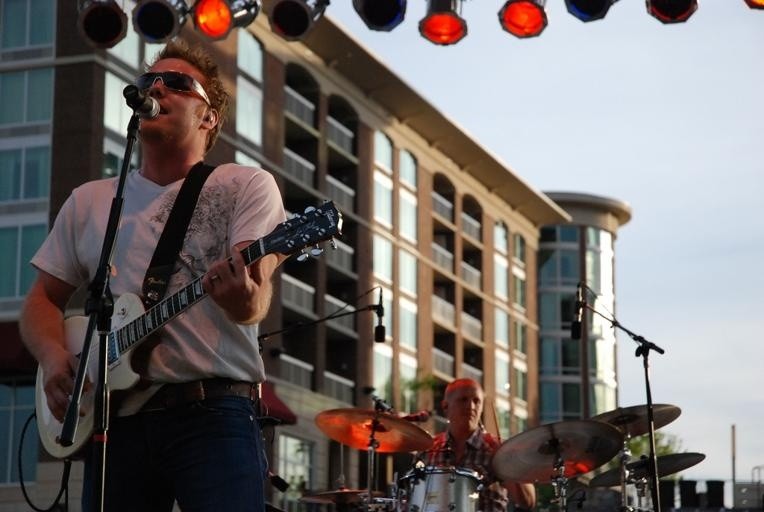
[314,408,433,455]
[589,453,698,487]
[593,403,681,440]
[492,421,623,482]
[299,489,385,504]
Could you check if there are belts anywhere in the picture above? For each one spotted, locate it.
[142,379,255,411]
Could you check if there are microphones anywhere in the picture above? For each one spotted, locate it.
[362,386,392,412]
[122,84,161,121]
[571,282,583,339]
[402,410,430,422]
[374,287,386,343]
[640,454,649,460]
[268,472,290,492]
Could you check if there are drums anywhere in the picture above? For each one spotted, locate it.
[365,497,407,512]
[406,465,482,512]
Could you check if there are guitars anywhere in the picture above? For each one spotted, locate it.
[35,198,344,458]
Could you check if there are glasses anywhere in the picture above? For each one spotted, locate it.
[134,71,211,107]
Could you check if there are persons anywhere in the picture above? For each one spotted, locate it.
[408,379,536,511]
[17,40,284,511]
